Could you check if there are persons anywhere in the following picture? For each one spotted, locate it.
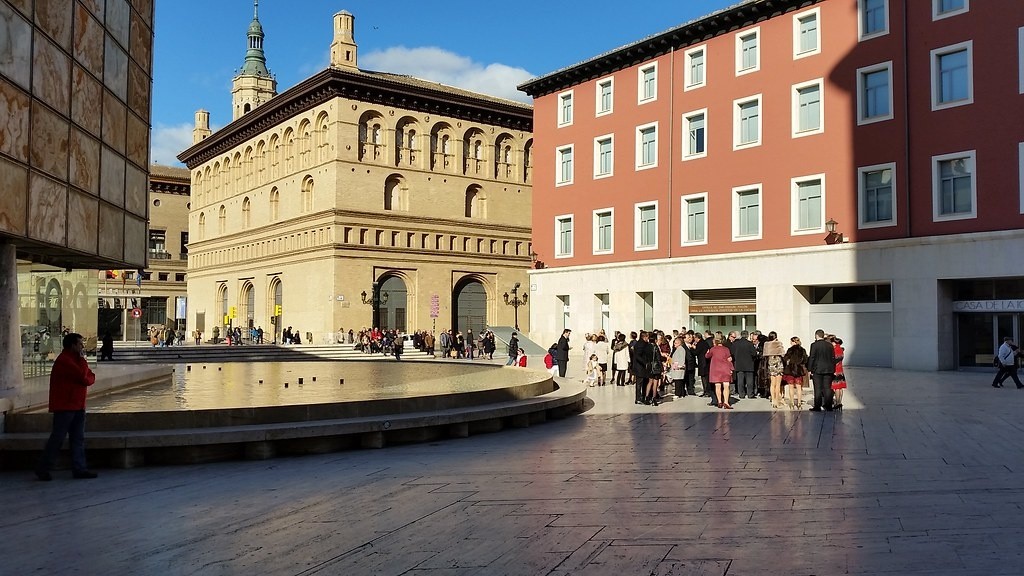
[62,326,69,335]
[22,328,28,354]
[35,325,51,351]
[37,333,98,480]
[338,327,495,359]
[549,329,573,377]
[585,327,847,413]
[281,326,301,345]
[507,332,526,367]
[148,326,159,347]
[194,329,201,345]
[212,326,220,344]
[226,326,243,346]
[158,324,168,346]
[252,326,263,343]
[992,337,1024,389]
[166,327,175,346]
[176,332,182,346]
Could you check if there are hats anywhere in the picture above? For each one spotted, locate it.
[1004,337,1013,341]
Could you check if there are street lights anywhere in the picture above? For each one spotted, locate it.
[361,282,389,327]
[503,283,528,330]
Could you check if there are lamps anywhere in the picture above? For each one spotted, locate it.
[529,251,544,269]
[379,292,389,305]
[361,291,373,304]
[826,217,843,244]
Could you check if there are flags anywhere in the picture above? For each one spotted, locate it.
[121,271,125,284]
[109,270,118,277]
[137,274,141,285]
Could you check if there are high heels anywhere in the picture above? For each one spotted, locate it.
[833,404,843,411]
[791,403,795,409]
[645,397,650,405]
[651,397,658,406]
[772,401,780,408]
[718,402,724,408]
[797,403,802,410]
[723,403,733,409]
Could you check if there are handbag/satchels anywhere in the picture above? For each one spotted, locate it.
[993,355,1002,367]
[671,366,684,380]
[653,362,661,374]
[451,349,458,356]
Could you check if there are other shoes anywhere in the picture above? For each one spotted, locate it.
[72,470,98,479]
[635,400,643,405]
[749,396,756,399]
[992,383,999,388]
[810,408,821,412]
[34,468,52,481]
[740,397,744,399]
[827,408,832,411]
[707,403,718,406]
